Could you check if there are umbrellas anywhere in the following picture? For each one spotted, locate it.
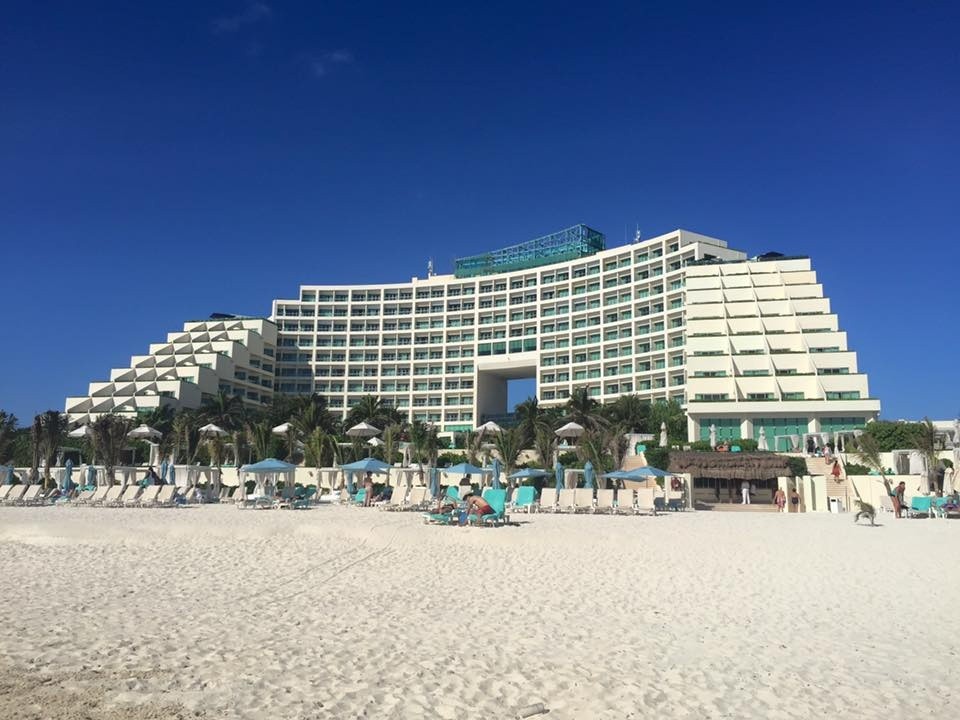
[553,421,585,446]
[623,465,674,489]
[601,468,649,501]
[890,481,905,518]
[472,420,508,444]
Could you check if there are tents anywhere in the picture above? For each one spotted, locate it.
[435,461,491,479]
[489,459,501,490]
[506,467,554,488]
[555,461,566,495]
[583,459,596,488]
[0,421,399,500]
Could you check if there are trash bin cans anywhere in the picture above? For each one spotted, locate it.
[830,497,843,512]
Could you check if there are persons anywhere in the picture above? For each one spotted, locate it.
[429,474,494,527]
[790,488,802,512]
[772,487,786,512]
[947,489,960,510]
[806,437,833,464]
[363,471,373,506]
[740,480,751,504]
[671,477,681,491]
[831,460,842,483]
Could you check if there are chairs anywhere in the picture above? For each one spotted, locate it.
[909,492,960,520]
[0,479,351,512]
[354,484,687,529]
